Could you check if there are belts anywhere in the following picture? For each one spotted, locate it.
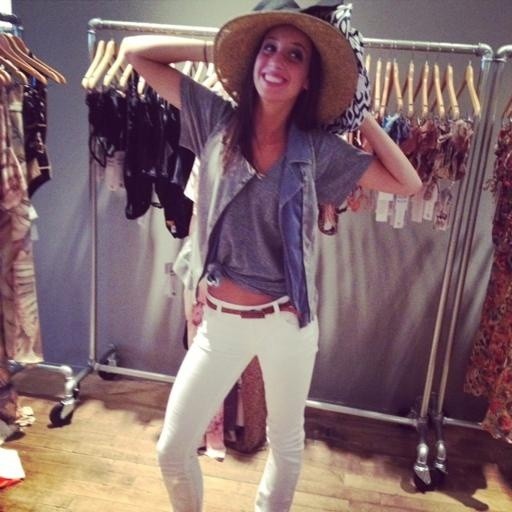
[205,296,302,319]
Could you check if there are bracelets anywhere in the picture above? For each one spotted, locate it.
[204,43,208,66]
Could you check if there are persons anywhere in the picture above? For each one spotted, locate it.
[122,11,422,511]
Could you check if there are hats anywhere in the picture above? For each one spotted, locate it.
[213,10,357,128]
